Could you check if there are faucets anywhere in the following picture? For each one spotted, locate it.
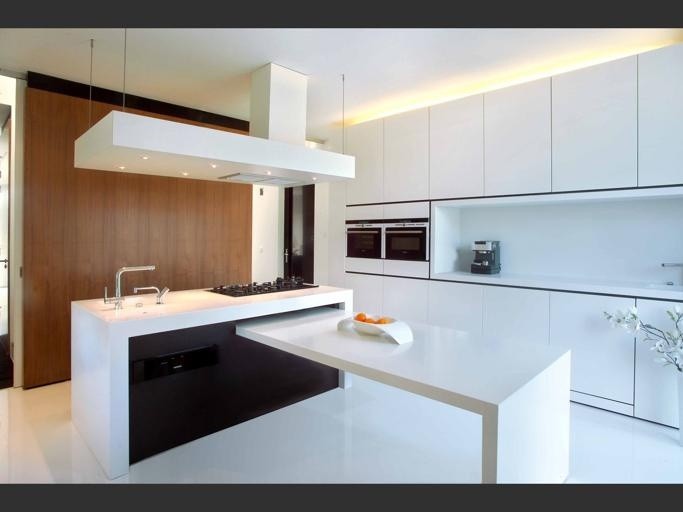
[134,285,160,294]
[116,265,155,308]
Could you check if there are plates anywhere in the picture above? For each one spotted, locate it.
[338,316,414,345]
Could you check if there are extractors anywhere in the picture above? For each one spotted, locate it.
[220,172,305,187]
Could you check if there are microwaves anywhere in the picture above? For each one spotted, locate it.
[382,223,430,261]
[345,223,383,261]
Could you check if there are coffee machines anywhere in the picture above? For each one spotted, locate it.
[471,241,502,273]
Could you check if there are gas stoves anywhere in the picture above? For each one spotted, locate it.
[213,275,303,296]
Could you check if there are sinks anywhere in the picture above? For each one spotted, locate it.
[113,296,164,307]
